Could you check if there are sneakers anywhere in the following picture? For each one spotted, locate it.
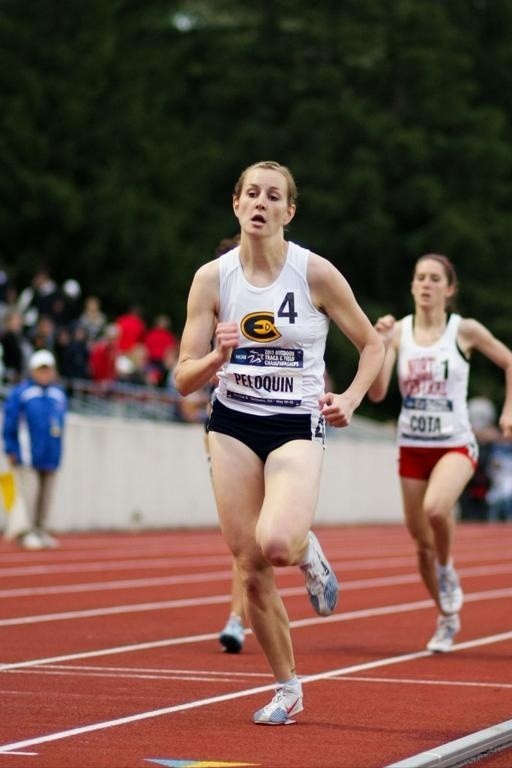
[252,685,304,725]
[426,614,460,653]
[21,533,60,552]
[434,560,463,614]
[219,621,244,653]
[300,532,338,617]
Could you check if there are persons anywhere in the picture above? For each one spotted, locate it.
[0,264,221,427]
[369,251,511,654]
[455,385,510,521]
[0,346,69,551]
[173,160,388,729]
[207,233,249,655]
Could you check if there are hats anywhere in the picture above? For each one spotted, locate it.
[30,349,55,370]
